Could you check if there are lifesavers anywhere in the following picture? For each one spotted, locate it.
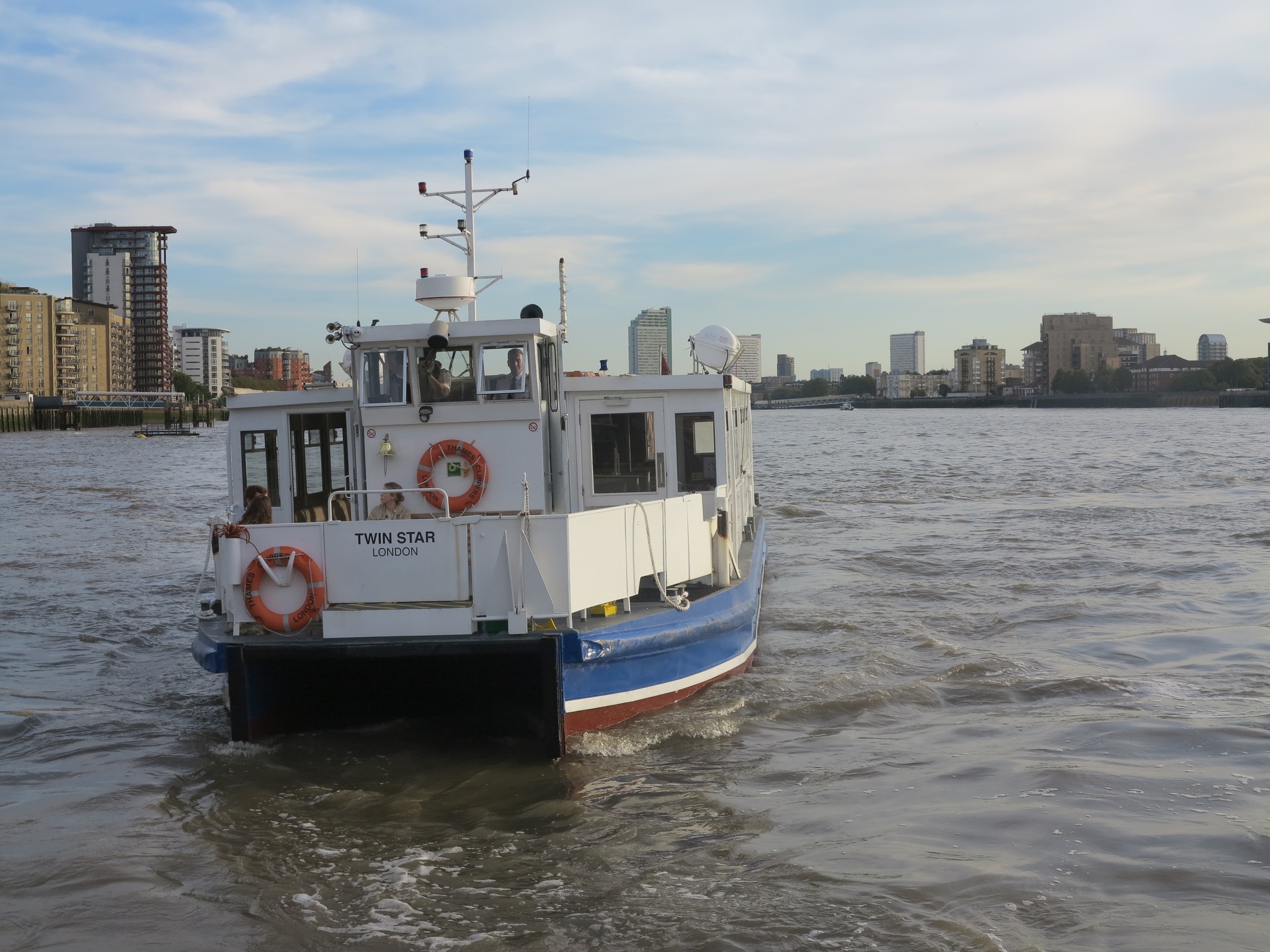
[417,439,487,511]
[242,546,323,633]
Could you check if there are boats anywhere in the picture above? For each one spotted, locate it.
[840,404,855,411]
[188,148,767,755]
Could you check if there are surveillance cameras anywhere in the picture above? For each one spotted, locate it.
[352,327,360,336]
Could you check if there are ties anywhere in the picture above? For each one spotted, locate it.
[507,377,516,400]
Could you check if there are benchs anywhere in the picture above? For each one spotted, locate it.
[593,466,656,493]
[295,499,352,523]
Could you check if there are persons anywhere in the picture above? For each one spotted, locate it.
[233,485,273,525]
[387,353,451,403]
[495,348,541,400]
[366,482,411,520]
